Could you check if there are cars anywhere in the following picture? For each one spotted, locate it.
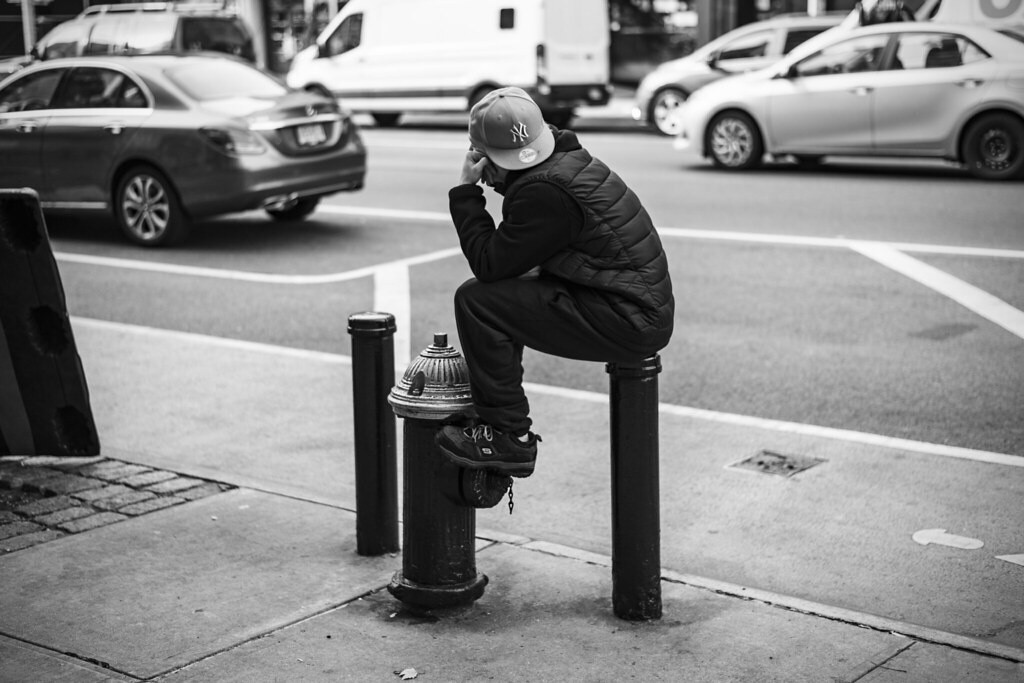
[682,23,1023,182]
[1,51,370,249]
[638,11,871,138]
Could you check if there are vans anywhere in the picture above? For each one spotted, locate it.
[286,0,614,129]
[31,1,264,80]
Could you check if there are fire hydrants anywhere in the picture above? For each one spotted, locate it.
[389,330,515,616]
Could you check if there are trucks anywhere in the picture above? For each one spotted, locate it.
[843,0,1024,34]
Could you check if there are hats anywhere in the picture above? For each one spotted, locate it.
[468,85,556,170]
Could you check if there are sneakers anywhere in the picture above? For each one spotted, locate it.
[434,423,542,478]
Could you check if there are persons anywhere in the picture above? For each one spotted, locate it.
[436,86,675,478]
